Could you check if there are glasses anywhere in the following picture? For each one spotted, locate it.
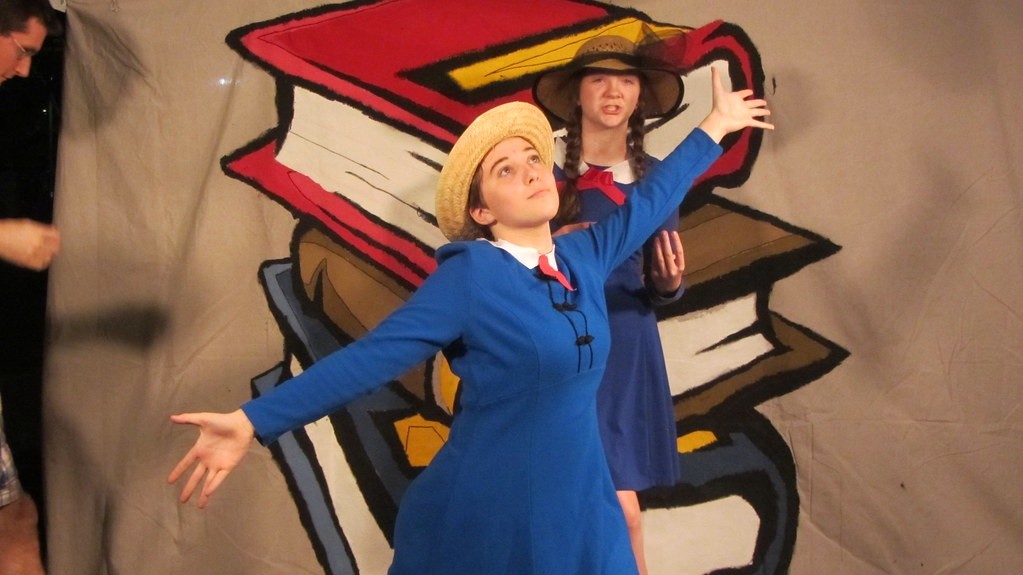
[10,33,32,63]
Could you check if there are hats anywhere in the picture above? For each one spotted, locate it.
[436,101,554,243]
[532,35,684,126]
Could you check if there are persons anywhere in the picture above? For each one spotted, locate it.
[533,36,686,575]
[0,0,65,575]
[166,67,777,575]
